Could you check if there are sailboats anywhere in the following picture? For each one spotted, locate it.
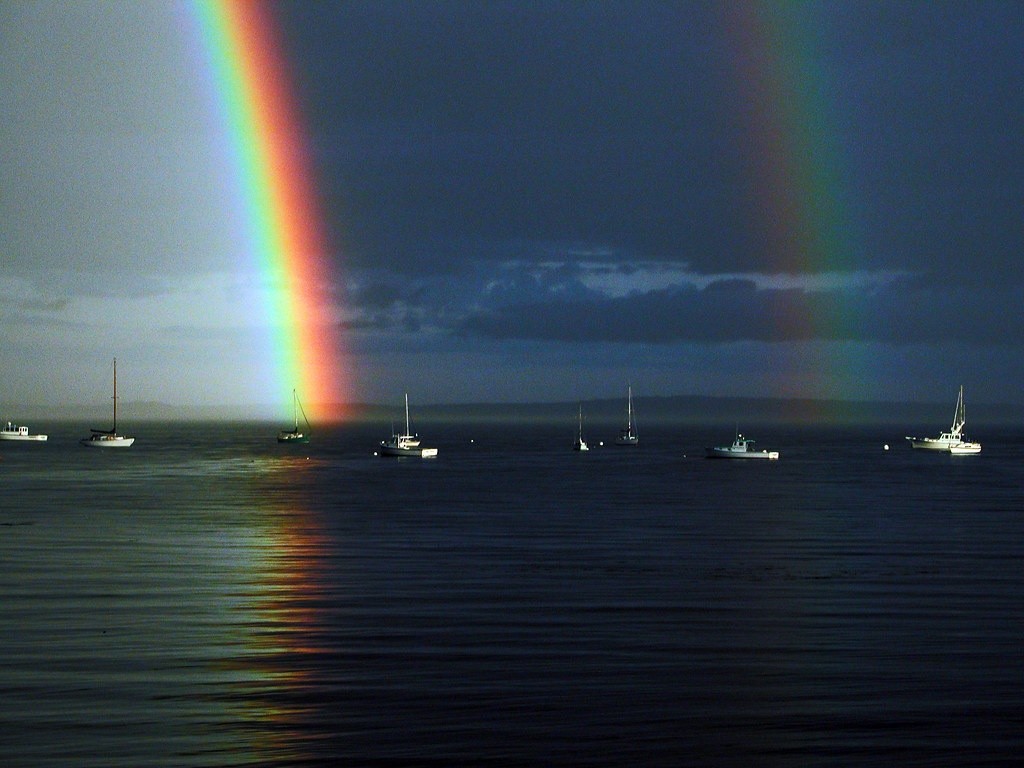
[616,386,640,446]
[572,403,589,451]
[79,355,135,447]
[948,385,982,454]
[276,390,314,444]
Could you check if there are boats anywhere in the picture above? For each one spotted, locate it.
[713,434,779,461]
[905,422,965,451]
[375,392,439,458]
[0,422,48,442]
